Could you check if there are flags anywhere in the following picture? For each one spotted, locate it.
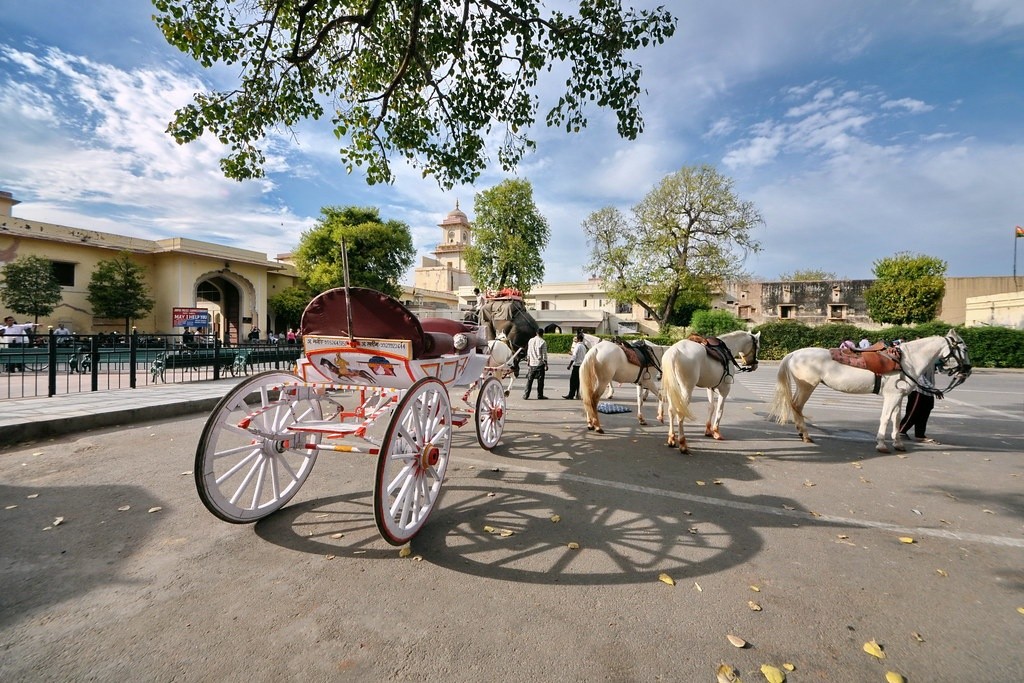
[1016,226,1024,237]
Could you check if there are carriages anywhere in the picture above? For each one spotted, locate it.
[192,233,525,546]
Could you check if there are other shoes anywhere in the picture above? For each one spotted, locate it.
[897,433,912,442]
[920,438,940,445]
[522,394,527,400]
[562,395,573,399]
[538,396,548,400]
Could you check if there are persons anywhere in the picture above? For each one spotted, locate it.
[899,364,944,442]
[279,330,286,340]
[894,337,906,345]
[561,333,587,400]
[857,334,872,349]
[24,320,33,348]
[194,327,206,343]
[248,325,261,340]
[14,321,29,372]
[295,328,302,336]
[3,316,43,373]
[521,328,549,400]
[286,328,297,344]
[53,323,70,336]
[840,335,856,349]
[267,330,278,344]
[182,326,194,342]
[474,288,485,322]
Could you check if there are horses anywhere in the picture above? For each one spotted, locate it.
[466,329,518,410]
[568,328,672,434]
[656,330,761,455]
[766,328,972,454]
